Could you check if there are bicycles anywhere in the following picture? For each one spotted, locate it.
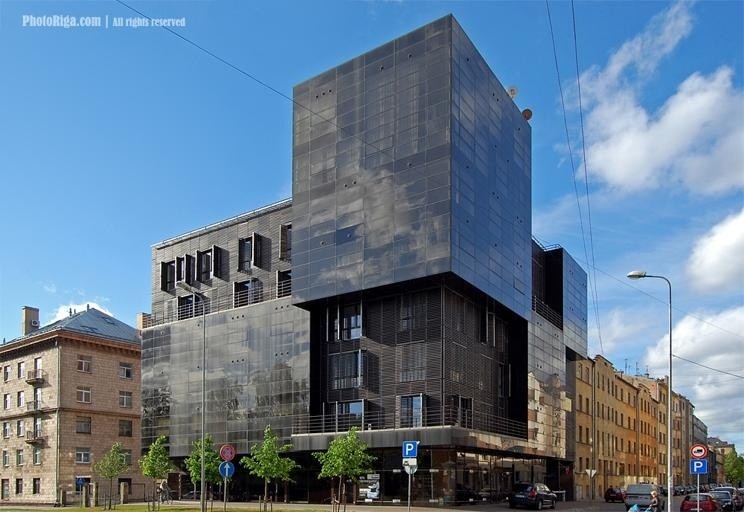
[158,489,173,505]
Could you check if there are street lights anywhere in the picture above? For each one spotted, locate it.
[175,280,206,512]
[626,269,673,512]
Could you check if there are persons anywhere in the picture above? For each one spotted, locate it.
[650,491,661,512]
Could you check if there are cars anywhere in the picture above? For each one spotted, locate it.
[505,480,558,511]
[479,487,501,502]
[604,487,625,502]
[455,483,478,506]
[663,484,710,497]
[680,487,744,512]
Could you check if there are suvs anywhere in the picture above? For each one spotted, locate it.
[624,484,665,511]
